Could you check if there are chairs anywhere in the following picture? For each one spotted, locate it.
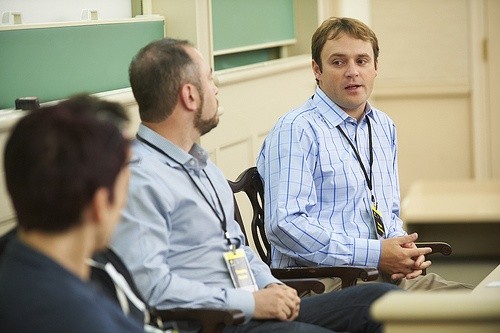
[227,167,452,288]
[95,248,325,333]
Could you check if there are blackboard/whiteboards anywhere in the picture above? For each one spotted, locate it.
[208,0,298,57]
[0,15,167,114]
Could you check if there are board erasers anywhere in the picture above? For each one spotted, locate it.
[15,97,41,113]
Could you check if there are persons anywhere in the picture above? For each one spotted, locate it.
[256,17,475,299]
[106,37,401,333]
[0,93,152,333]
